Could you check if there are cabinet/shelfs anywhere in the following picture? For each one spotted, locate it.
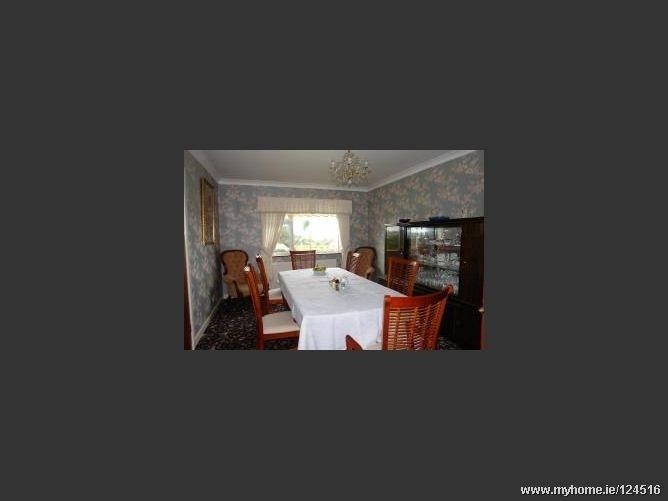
[383,216,484,350]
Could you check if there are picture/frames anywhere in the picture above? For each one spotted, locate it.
[201,176,215,245]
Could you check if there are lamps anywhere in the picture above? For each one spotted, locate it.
[329,150,373,189]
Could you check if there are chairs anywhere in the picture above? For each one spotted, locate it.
[243,247,453,350]
[220,250,264,312]
